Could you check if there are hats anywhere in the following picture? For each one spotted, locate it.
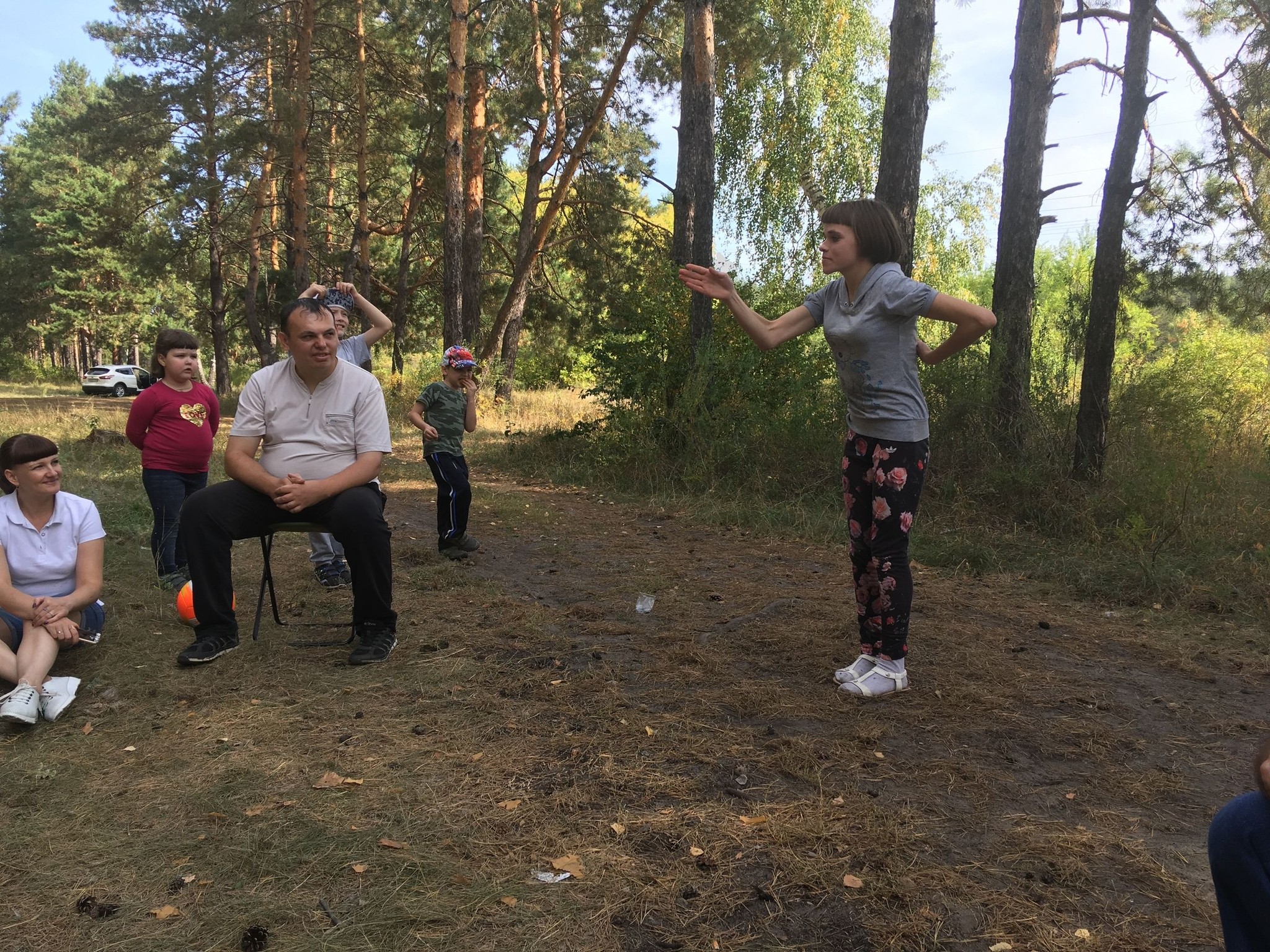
[442,345,478,369]
[314,288,354,313]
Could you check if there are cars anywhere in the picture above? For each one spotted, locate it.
[82,364,163,398]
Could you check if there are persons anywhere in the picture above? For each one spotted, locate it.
[126,330,221,593]
[176,298,392,666]
[0,433,107,727]
[678,199,998,697]
[408,346,479,560]
[297,282,394,590]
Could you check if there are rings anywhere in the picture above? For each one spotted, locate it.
[70,625,75,630]
[56,630,62,633]
[44,608,49,613]
[49,612,54,617]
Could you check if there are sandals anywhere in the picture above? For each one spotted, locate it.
[833,654,879,685]
[838,666,911,699]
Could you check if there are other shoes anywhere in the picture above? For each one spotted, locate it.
[161,572,187,592]
[179,565,192,581]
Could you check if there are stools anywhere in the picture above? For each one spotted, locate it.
[251,523,357,647]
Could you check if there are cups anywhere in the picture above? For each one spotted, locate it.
[636,592,656,612]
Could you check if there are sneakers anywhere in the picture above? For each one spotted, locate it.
[313,564,341,589]
[177,631,240,665]
[0,678,39,724]
[456,534,479,551]
[333,561,352,585]
[439,546,468,561]
[348,628,398,664]
[38,676,81,723]
